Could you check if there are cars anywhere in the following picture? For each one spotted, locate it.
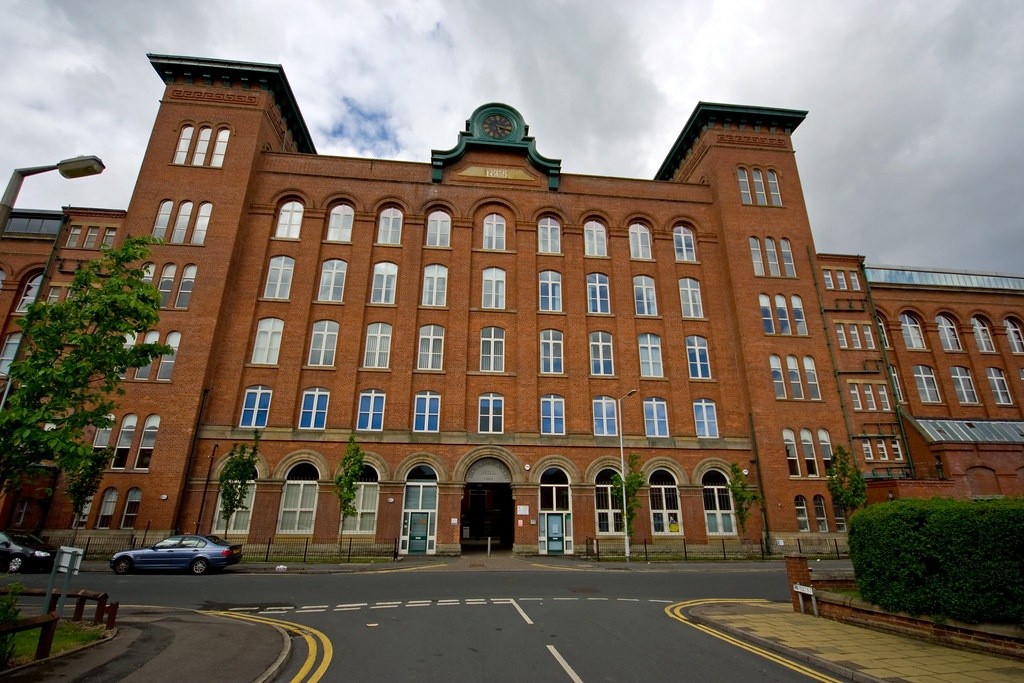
[0,530,60,575]
[109,534,243,576]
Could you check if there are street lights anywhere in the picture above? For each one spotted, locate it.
[0,153,105,236]
[618,390,637,560]
